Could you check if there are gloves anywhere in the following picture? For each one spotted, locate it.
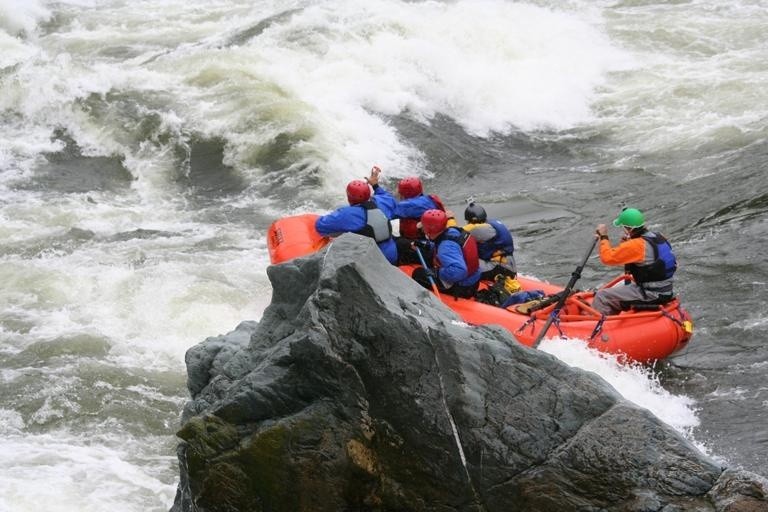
[409,240,430,250]
[420,268,438,285]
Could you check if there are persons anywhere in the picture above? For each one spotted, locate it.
[591,207,673,315]
[411,209,481,299]
[314,167,400,265]
[391,176,447,265]
[444,202,517,282]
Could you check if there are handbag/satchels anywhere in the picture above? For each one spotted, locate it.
[488,283,510,305]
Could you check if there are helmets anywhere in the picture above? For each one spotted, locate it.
[613,207,646,227]
[465,203,487,222]
[399,176,422,198]
[422,209,448,234]
[347,180,370,205]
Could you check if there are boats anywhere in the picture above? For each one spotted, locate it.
[267,215,692,370]
